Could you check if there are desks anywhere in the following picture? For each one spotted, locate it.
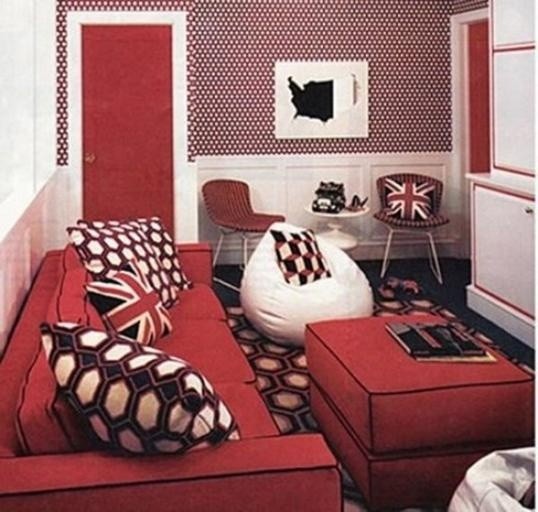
[305,202,369,251]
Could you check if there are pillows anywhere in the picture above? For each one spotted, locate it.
[39,321,243,455]
[66,216,193,311]
[82,258,174,346]
[270,228,332,287]
[381,175,435,221]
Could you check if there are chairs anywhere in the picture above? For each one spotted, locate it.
[239,220,373,346]
[373,173,450,286]
[202,179,285,293]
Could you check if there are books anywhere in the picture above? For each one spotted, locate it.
[416,351,499,364]
[384,320,486,357]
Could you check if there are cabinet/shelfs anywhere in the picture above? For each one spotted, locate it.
[464,0,536,351]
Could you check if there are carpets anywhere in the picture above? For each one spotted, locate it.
[224,277,536,512]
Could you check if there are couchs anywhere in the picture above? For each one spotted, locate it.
[0,241,347,511]
